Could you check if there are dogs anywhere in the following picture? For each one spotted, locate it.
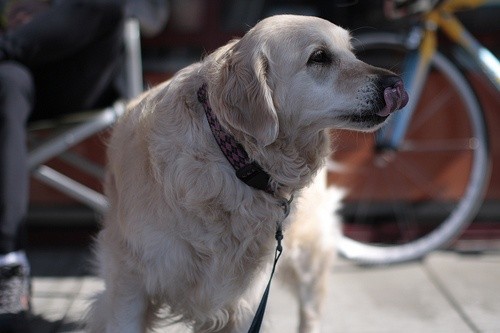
[77,14,411,333]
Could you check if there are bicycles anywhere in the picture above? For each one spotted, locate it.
[334,0,500,268]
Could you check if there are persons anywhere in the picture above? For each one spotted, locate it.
[0,0,170,333]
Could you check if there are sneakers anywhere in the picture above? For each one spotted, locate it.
[0,253,31,326]
